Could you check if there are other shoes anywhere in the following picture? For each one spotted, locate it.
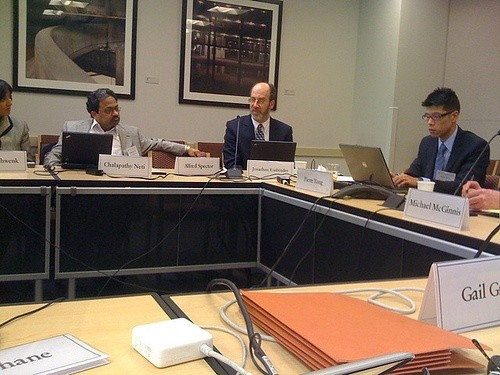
[230,269,252,287]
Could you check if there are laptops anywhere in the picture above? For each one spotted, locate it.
[304,351,415,375]
[338,143,417,192]
[60,131,113,169]
[250,140,297,163]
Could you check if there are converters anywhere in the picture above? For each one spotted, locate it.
[132,317,214,368]
[277,176,290,184]
[86,168,103,176]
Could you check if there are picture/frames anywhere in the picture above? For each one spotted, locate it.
[11,0,139,100]
[179,0,283,110]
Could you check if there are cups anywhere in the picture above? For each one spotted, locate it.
[295,161,306,175]
[417,181,436,192]
[327,164,338,181]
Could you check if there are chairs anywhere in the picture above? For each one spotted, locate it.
[149,140,225,168]
[34,134,59,164]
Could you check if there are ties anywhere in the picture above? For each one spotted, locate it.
[432,143,446,181]
[255,124,264,141]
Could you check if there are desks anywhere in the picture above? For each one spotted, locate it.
[0,162,500,375]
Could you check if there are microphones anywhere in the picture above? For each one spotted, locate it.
[225,115,244,178]
[454,130,500,195]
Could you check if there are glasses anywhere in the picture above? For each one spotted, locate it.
[98,106,122,114]
[421,110,456,121]
[248,98,270,104]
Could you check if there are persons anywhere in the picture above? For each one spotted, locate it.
[461,180,500,209]
[223,82,293,170]
[0,79,34,161]
[390,88,490,197]
[42,88,201,165]
